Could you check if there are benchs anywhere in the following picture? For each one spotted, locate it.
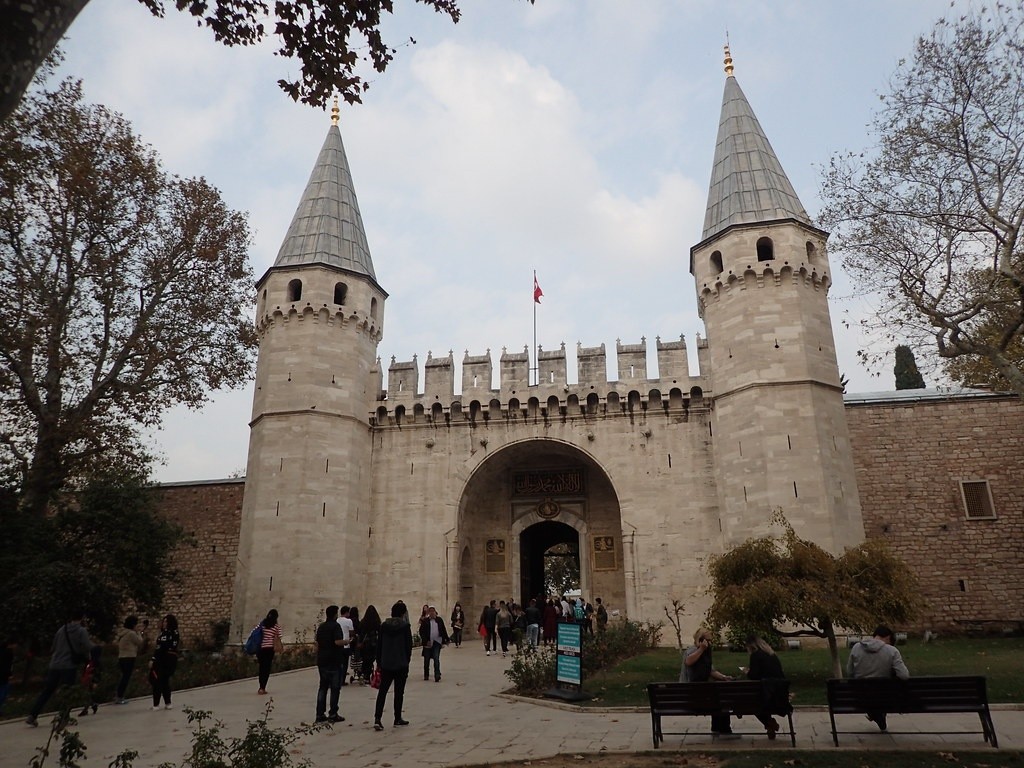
[646,678,796,748]
[825,675,999,748]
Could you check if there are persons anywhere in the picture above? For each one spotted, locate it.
[418,605,449,682]
[336,605,381,685]
[846,626,910,731]
[314,606,351,722]
[26,606,104,727]
[396,600,409,623]
[679,628,741,739]
[478,596,594,657]
[594,598,607,641]
[149,614,179,710]
[0,639,21,706]
[451,603,464,648]
[114,615,148,705]
[251,609,282,695]
[78,646,103,716]
[742,635,785,740]
[374,603,409,730]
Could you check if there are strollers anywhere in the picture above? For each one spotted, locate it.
[347,646,376,685]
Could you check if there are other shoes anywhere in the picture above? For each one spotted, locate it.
[424,673,429,681]
[115,697,127,703]
[395,717,410,728]
[78,709,89,717]
[26,715,39,726]
[503,651,505,655]
[487,651,491,657]
[434,675,440,682]
[374,719,384,729]
[328,713,345,722]
[316,714,327,723]
[91,703,98,713]
[165,702,172,710]
[258,687,266,695]
[710,730,741,739]
[149,705,160,711]
[768,720,780,739]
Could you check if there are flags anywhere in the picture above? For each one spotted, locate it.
[533,277,543,304]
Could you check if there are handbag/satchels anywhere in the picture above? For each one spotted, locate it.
[370,669,381,690]
[72,652,86,666]
[244,624,263,655]
[480,624,487,636]
